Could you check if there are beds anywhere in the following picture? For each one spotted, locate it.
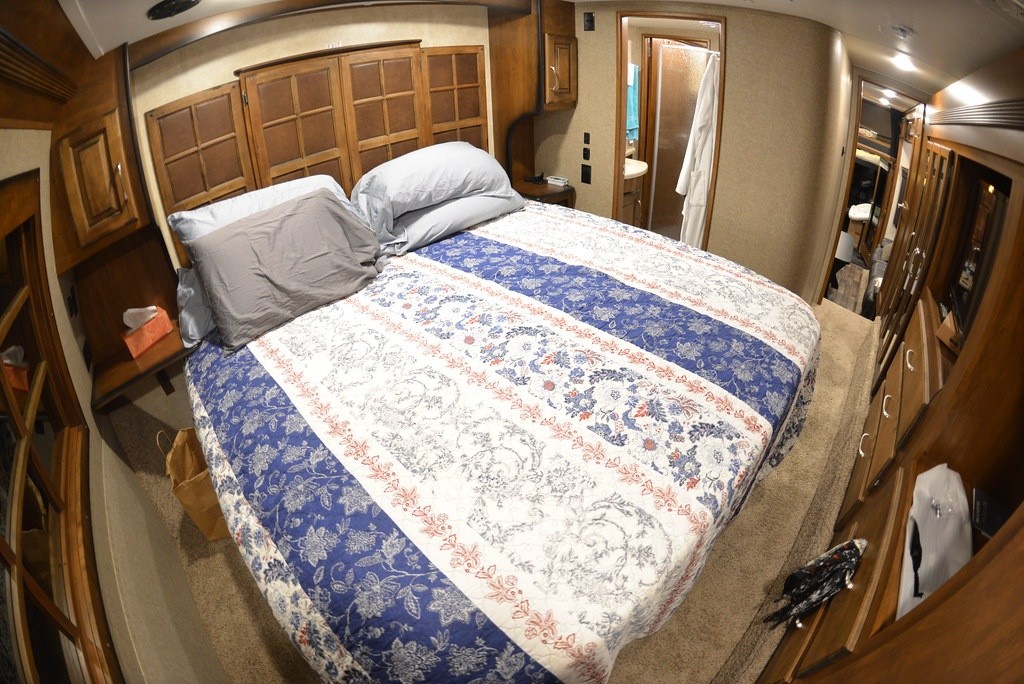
[184,202,824,684]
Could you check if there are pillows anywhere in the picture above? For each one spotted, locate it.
[185,194,380,351]
[167,175,348,281]
[358,139,511,245]
[395,190,526,265]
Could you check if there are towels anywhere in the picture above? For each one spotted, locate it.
[626,64,639,140]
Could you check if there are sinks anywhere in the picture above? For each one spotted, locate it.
[624,157,648,179]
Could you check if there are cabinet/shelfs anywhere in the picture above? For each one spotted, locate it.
[622,176,645,228]
[50,46,186,411]
[753,125,1024,684]
[486,0,579,209]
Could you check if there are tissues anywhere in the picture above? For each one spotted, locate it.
[119,305,174,360]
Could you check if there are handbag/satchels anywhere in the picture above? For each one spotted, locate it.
[157,427,234,540]
[893,464,972,619]
